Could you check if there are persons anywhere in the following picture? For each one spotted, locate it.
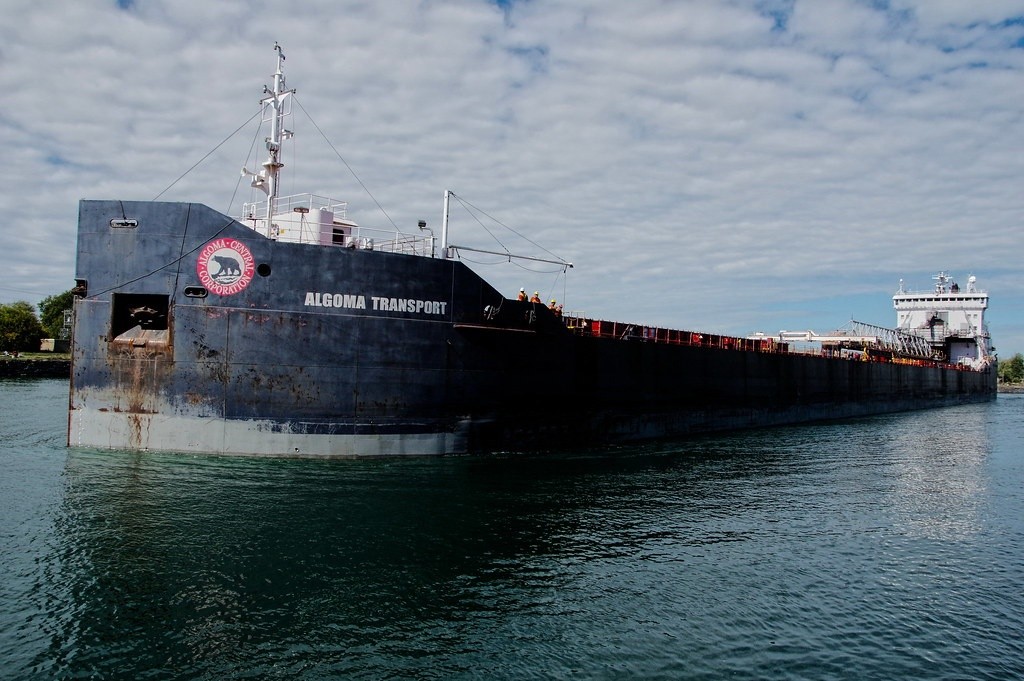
[518,288,528,302]
[530,291,542,303]
[547,298,556,313]
[555,304,563,314]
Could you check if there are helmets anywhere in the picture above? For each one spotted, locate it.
[520,288,525,291]
[534,292,538,295]
[551,299,556,302]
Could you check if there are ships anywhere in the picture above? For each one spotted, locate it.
[67,41,998,458]
